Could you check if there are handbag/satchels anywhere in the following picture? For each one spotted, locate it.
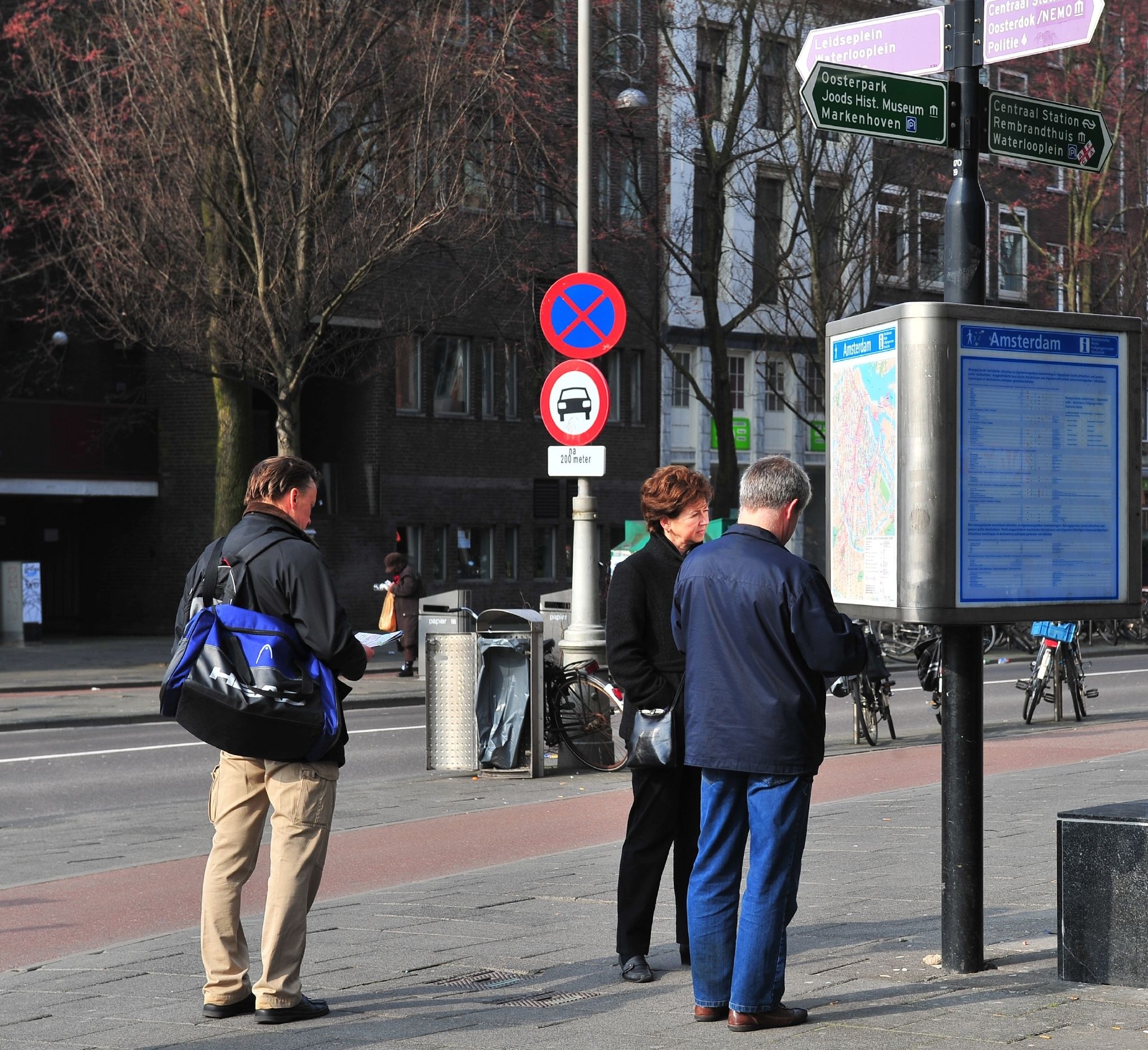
[913,634,940,692]
[378,581,398,633]
[158,533,348,762]
[627,708,677,769]
[856,633,892,684]
[829,676,850,698]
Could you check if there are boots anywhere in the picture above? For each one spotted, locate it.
[397,661,413,677]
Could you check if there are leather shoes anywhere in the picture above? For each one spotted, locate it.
[678,943,691,965]
[202,991,255,1018]
[617,953,651,982]
[728,1001,807,1032]
[694,1004,729,1022]
[254,990,330,1023]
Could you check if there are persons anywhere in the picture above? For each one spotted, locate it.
[173,454,377,1025]
[668,455,867,1033]
[603,469,722,984]
[381,552,427,678]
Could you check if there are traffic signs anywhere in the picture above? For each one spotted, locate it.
[984,90,1114,174]
[791,5,947,88]
[801,59,951,151]
[978,0,1107,66]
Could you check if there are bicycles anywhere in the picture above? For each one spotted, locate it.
[833,588,1148,748]
[450,607,633,773]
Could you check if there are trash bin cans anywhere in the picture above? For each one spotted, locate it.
[425,608,545,779]
[418,589,472,681]
[540,589,570,713]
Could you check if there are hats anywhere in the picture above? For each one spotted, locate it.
[383,553,403,573]
